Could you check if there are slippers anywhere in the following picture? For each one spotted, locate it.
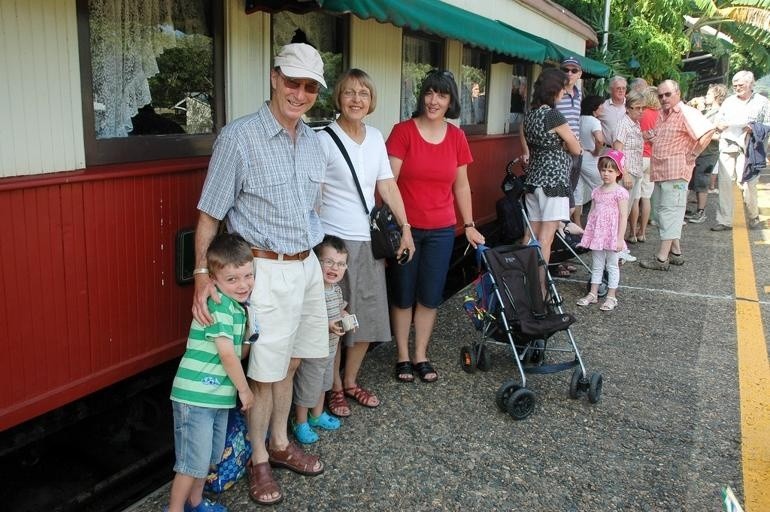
[397,361,414,382]
[187,499,228,512]
[290,417,320,444]
[309,411,340,430]
[415,361,438,382]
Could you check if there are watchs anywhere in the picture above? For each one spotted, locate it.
[465,222,475,228]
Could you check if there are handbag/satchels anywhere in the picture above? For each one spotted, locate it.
[322,126,409,262]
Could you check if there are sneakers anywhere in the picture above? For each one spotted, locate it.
[689,210,706,222]
[617,252,637,264]
[639,256,671,271]
[711,224,732,232]
[629,237,637,243]
[749,216,759,228]
[638,235,646,242]
[668,253,685,265]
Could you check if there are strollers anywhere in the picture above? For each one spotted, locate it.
[462,185,604,421]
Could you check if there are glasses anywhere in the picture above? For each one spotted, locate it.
[615,86,627,90]
[341,89,371,98]
[562,67,581,74]
[276,69,321,93]
[657,91,673,99]
[319,257,348,269]
[427,67,454,80]
[629,106,647,111]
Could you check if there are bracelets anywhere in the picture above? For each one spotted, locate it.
[399,223,411,228]
[192,267,210,275]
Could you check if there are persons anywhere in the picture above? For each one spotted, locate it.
[293,233,355,446]
[576,149,630,311]
[712,70,769,232]
[639,79,714,271]
[191,43,327,505]
[165,232,253,511]
[554,57,583,145]
[625,77,662,245]
[599,76,627,147]
[572,95,600,225]
[687,84,725,224]
[317,68,415,419]
[614,92,643,263]
[521,69,584,302]
[386,69,485,382]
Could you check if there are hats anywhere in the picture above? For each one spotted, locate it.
[274,43,328,89]
[600,149,626,180]
[561,56,582,67]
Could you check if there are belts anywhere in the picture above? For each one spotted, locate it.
[603,143,613,148]
[253,248,310,261]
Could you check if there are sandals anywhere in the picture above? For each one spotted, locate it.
[268,441,325,475]
[329,389,352,417]
[247,457,283,505]
[563,262,578,273]
[550,264,570,278]
[600,296,618,311]
[344,383,380,408]
[576,292,598,306]
